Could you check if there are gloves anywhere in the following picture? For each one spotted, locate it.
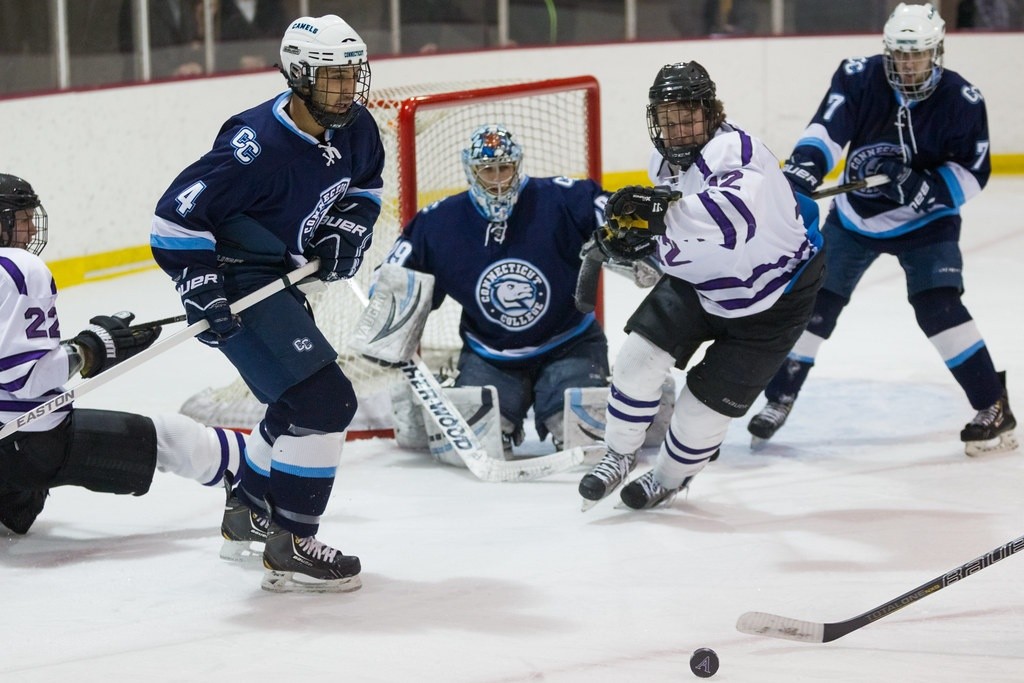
[605,186,681,239]
[174,266,244,347]
[595,227,657,262]
[873,157,957,212]
[302,194,382,281]
[69,311,161,378]
[780,153,823,198]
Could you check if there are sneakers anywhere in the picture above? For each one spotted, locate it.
[262,520,362,594]
[220,469,274,560]
[960,373,1019,457]
[746,390,795,450]
[615,467,694,510]
[579,444,638,512]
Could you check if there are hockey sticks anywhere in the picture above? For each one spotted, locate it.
[732,538,1024,644]
[574,172,891,315]
[344,276,586,485]
[60,314,188,346]
[0,257,321,442]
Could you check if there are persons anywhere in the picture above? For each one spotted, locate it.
[0,173,250,535]
[746,3,1020,461]
[150,14,386,595]
[118,0,756,82]
[368,123,616,462]
[578,59,827,514]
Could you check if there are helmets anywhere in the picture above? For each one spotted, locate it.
[462,124,524,220]
[0,173,40,231]
[649,60,718,162]
[280,14,367,129]
[881,2,946,98]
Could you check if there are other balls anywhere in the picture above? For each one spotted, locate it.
[689,647,720,678]
